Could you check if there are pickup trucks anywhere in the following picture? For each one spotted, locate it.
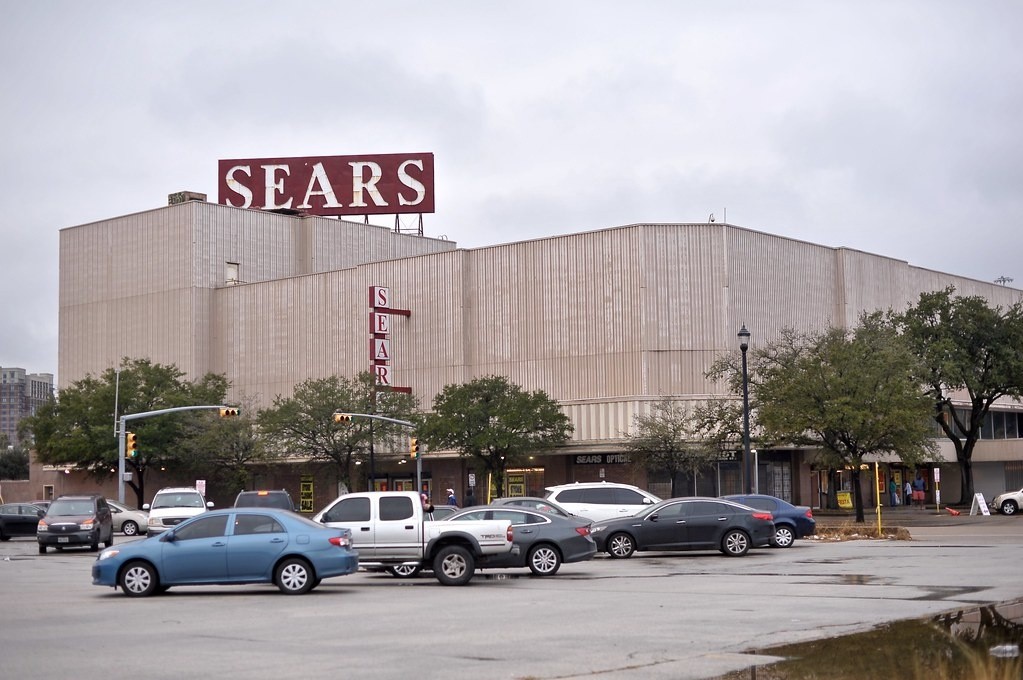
[254,491,520,586]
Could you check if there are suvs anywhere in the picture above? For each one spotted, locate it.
[535,481,664,522]
[143,486,214,538]
[234,487,298,528]
[36,493,113,554]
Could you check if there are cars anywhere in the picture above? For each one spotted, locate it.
[106,499,149,536]
[367,497,597,576]
[0,500,52,541]
[589,497,776,559]
[91,507,359,598]
[716,494,816,548]
[991,488,1023,516]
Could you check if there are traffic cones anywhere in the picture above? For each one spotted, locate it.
[945,507,960,516]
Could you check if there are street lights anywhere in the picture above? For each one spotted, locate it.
[737,321,751,494]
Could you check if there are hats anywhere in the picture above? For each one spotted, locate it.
[447,489,454,495]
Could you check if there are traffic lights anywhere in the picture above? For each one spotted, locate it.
[410,438,420,459]
[127,434,138,458]
[220,408,240,417]
[335,415,353,423]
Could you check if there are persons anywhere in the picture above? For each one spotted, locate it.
[911,473,926,510]
[421,494,435,513]
[890,477,898,507]
[904,481,912,505]
[447,489,456,505]
[463,488,476,508]
